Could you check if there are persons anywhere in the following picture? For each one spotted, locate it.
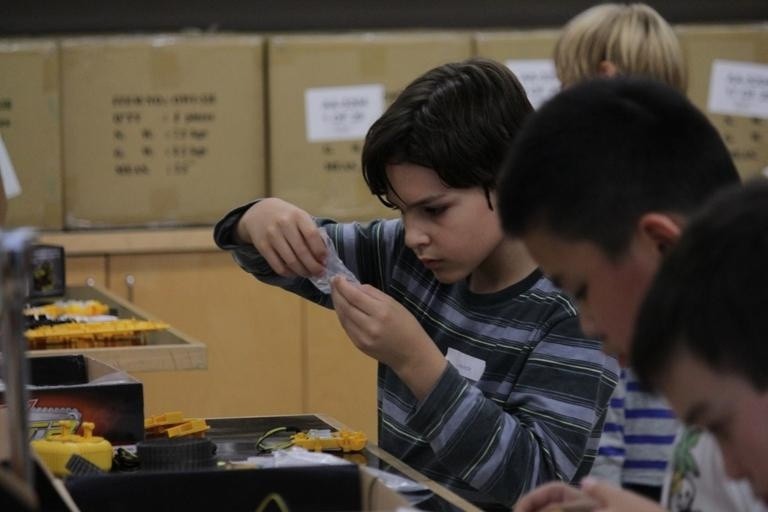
[553,3,687,99]
[631,172,766,510]
[213,55,622,511]
[496,72,742,510]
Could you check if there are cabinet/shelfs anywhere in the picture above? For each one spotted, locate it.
[38,230,377,441]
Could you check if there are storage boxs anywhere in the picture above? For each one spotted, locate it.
[0,354,145,464]
[0,25,768,233]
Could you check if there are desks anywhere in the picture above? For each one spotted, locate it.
[207,414,486,512]
[27,285,207,374]
